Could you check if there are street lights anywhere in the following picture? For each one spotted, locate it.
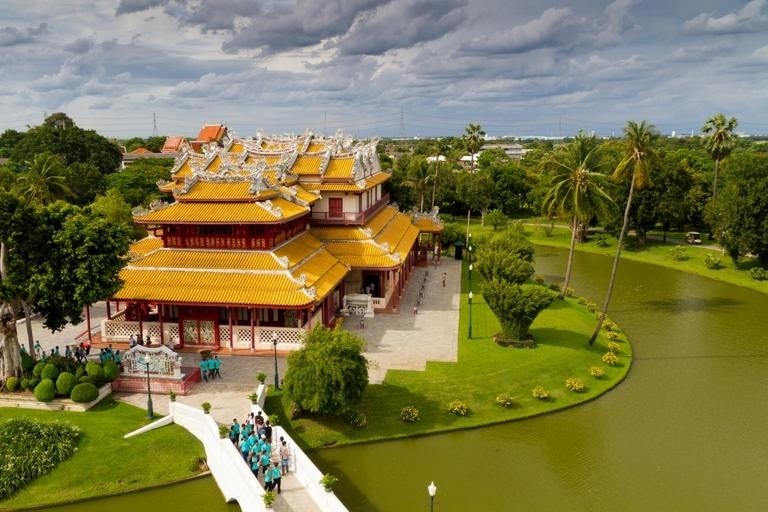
[468,233,473,339]
[144,351,155,419]
[273,334,279,389]
[427,480,437,512]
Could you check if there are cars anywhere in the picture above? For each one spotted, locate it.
[686,232,702,244]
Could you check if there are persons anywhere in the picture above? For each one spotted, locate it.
[215,356,221,376]
[362,282,375,295]
[199,358,209,382]
[168,338,175,351]
[35,341,42,360]
[136,334,143,346]
[207,356,216,379]
[229,410,289,495]
[21,344,26,352]
[99,345,121,366]
[145,337,152,346]
[42,342,91,363]
[442,273,446,286]
[129,335,135,348]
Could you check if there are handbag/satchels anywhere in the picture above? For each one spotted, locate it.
[282,461,288,466]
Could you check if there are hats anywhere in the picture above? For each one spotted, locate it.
[261,434,266,439]
